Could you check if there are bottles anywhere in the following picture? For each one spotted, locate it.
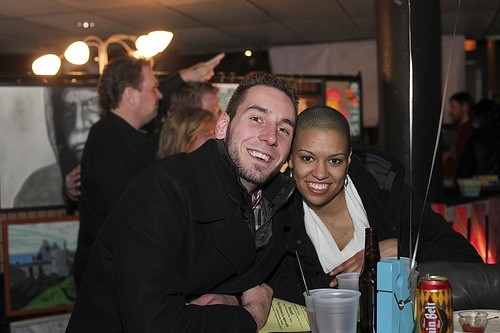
[357,228,381,333]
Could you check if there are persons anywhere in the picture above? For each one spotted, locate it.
[356,125,390,163]
[63,59,225,216]
[438,90,500,207]
[266,103,496,333]
[62,67,299,333]
[63,54,165,299]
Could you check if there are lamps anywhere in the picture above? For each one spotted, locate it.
[31,30,174,85]
[464,33,481,60]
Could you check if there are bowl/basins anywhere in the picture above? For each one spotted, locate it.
[457,178,483,196]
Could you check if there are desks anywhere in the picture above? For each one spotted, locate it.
[420,185,500,264]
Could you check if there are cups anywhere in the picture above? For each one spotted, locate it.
[458,311,489,333]
[303,289,335,333]
[336,272,361,323]
[311,290,362,333]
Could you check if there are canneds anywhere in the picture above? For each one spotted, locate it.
[415,273,454,333]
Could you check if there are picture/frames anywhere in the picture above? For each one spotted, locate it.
[2,216,80,318]
